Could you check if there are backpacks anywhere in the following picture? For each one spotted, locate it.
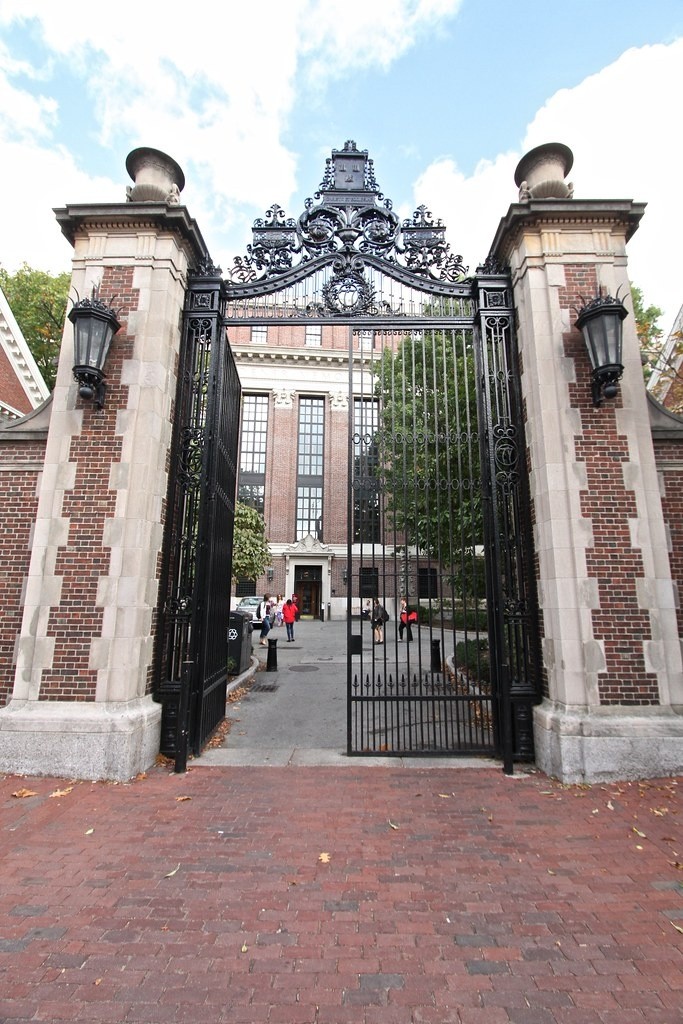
[257,601,266,619]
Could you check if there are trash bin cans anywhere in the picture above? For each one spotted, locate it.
[229,610,254,674]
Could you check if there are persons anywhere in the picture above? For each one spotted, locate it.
[397,597,414,643]
[370,596,384,645]
[361,599,371,620]
[259,593,299,645]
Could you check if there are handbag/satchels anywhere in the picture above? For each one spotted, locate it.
[381,610,389,622]
[400,610,418,625]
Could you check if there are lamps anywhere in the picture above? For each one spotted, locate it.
[267,569,273,582]
[342,567,348,585]
[569,283,630,408]
[64,283,125,408]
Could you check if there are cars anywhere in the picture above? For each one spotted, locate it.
[235,596,275,629]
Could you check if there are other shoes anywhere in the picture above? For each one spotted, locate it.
[291,639,295,641]
[259,638,268,645]
[398,639,403,642]
[287,640,291,642]
[409,641,413,643]
[374,639,384,645]
